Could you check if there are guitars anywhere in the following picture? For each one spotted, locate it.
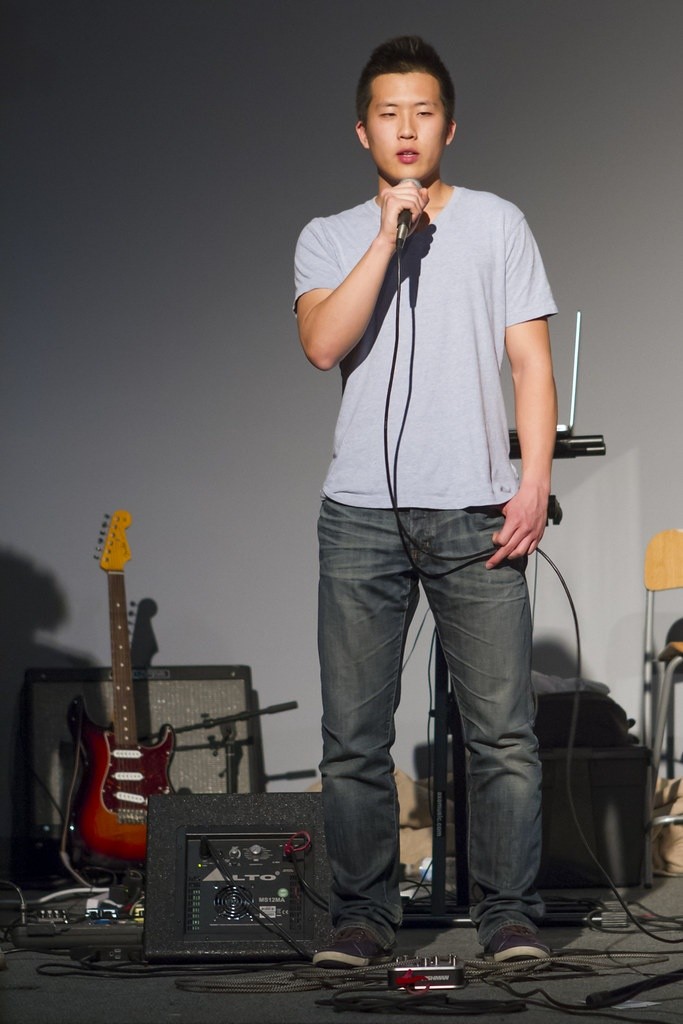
[56,508,178,876]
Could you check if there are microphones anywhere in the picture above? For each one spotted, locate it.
[396,179,423,249]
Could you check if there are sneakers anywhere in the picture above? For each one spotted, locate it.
[483,924,551,965]
[310,927,396,969]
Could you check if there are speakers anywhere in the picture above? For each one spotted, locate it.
[145,793,335,960]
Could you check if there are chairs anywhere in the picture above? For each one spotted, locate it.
[638,525,682,891]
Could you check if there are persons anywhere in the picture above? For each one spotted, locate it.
[289,34,561,971]
